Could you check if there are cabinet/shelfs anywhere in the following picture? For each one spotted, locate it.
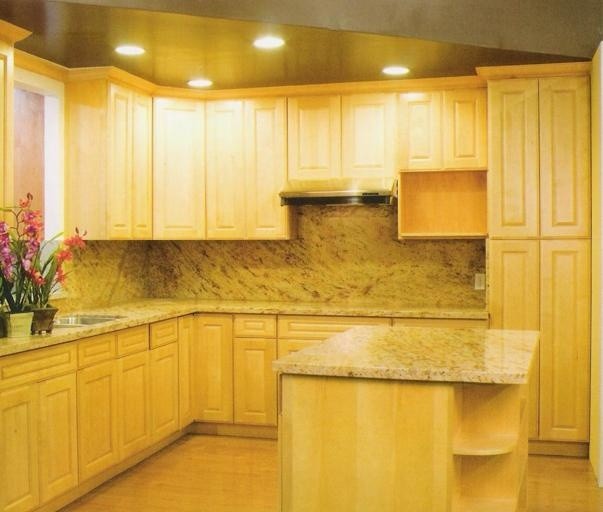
[153,83,205,240]
[272,326,488,510]
[1,310,79,511]
[150,310,189,455]
[205,85,298,240]
[392,309,488,327]
[77,309,150,498]
[276,310,391,360]
[475,62,590,239]
[189,309,278,440]
[14,47,70,241]
[279,80,398,206]
[70,66,153,240]
[485,240,592,460]
[1,19,33,237]
[398,75,488,240]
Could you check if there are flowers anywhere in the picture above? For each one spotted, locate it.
[0,194,87,308]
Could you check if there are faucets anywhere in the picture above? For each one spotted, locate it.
[49,283,68,294]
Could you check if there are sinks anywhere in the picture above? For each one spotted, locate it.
[53,314,127,326]
